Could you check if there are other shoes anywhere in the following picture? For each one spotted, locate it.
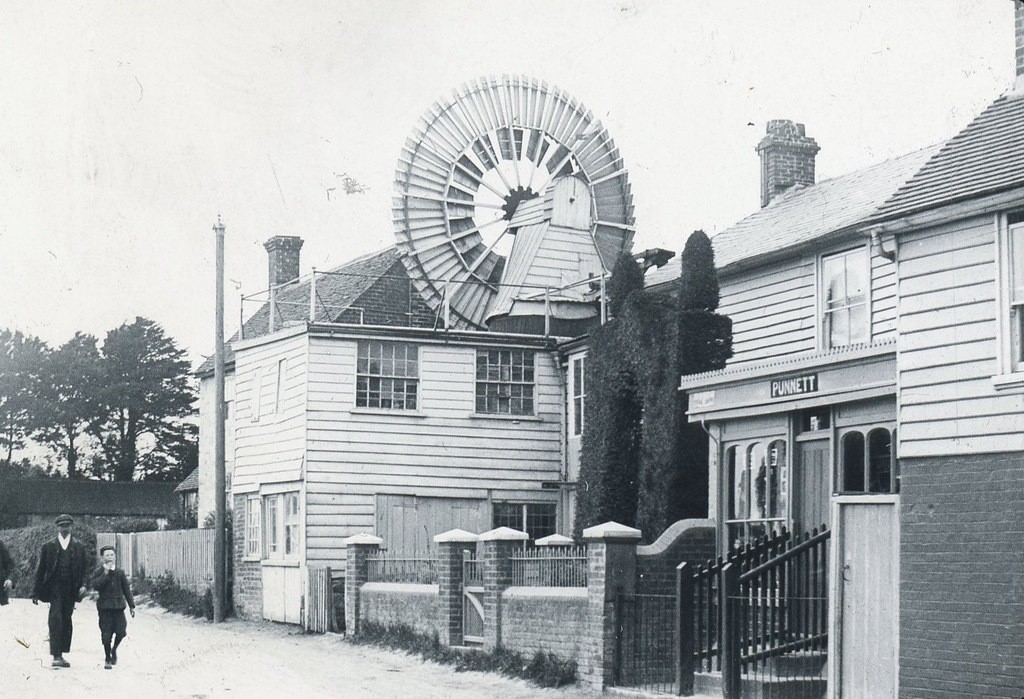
[104,657,112,669]
[52,657,70,667]
[110,649,117,665]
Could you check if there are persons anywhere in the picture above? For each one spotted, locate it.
[32,514,90,667]
[0,539,14,606]
[92,545,136,670]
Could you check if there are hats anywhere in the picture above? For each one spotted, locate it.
[55,514,74,526]
